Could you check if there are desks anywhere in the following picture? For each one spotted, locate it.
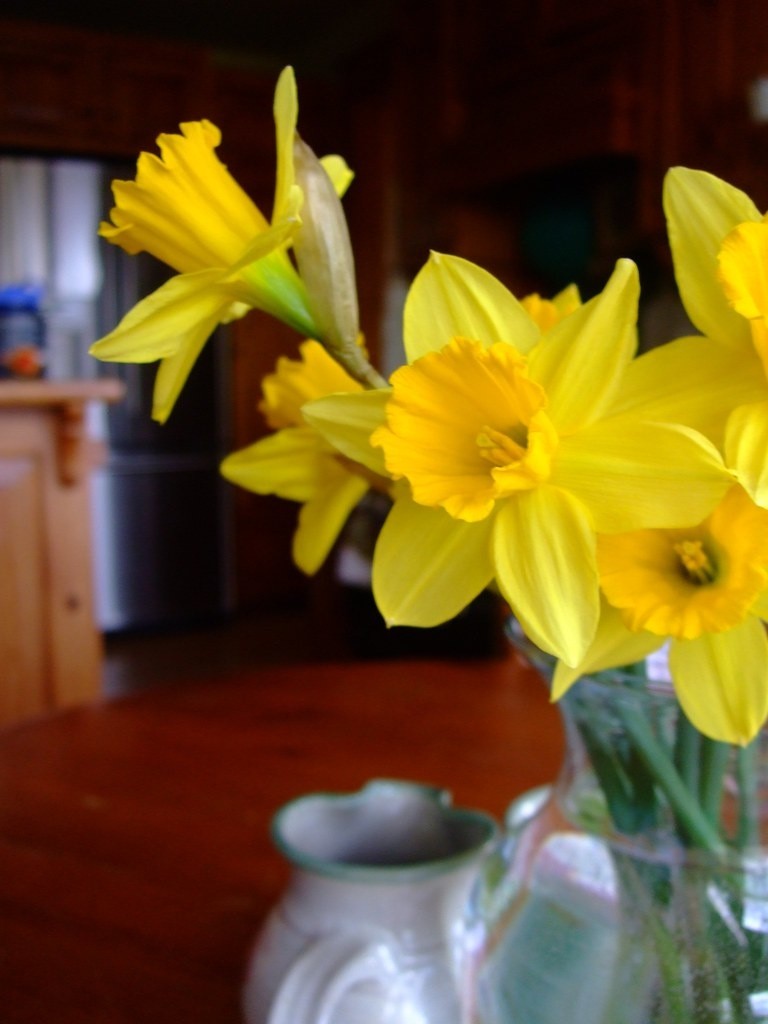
[0,664,768,1024]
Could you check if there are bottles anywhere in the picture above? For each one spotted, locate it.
[0,285,52,383]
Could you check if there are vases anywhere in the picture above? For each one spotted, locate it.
[241,779,503,1024]
[468,615,768,1024]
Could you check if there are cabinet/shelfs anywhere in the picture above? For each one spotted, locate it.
[0,379,125,726]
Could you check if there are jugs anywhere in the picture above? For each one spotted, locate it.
[242,775,497,1024]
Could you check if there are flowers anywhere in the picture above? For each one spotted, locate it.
[86,59,768,752]
